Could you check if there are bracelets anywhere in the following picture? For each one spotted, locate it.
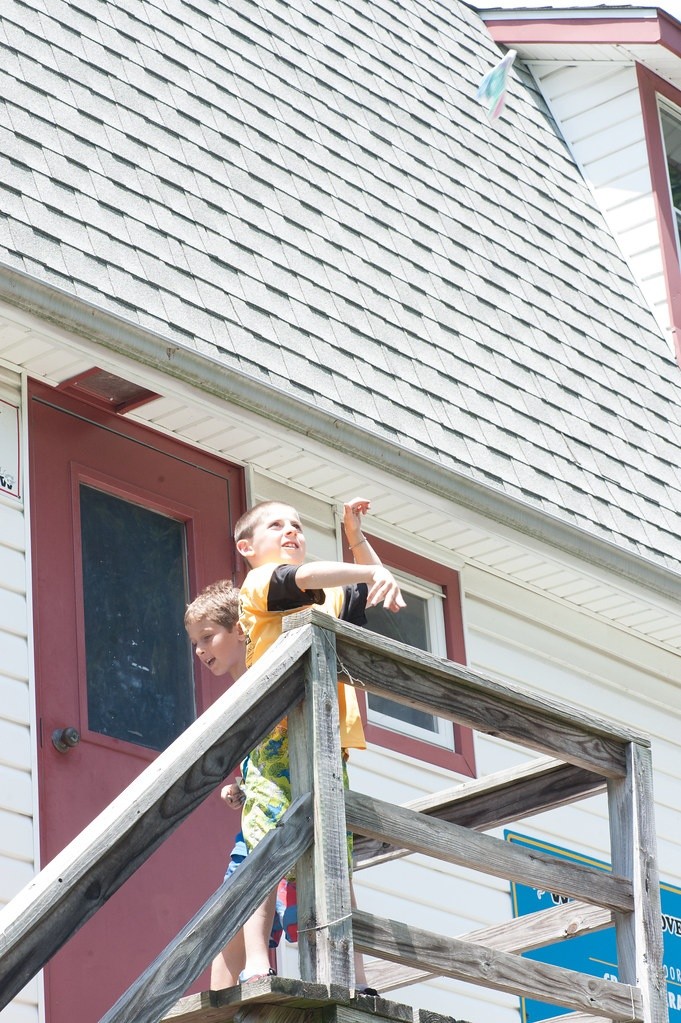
[348,537,367,551]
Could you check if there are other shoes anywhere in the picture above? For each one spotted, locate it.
[238,967,276,984]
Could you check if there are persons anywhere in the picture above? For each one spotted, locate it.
[183,576,297,991]
[239,495,407,981]
